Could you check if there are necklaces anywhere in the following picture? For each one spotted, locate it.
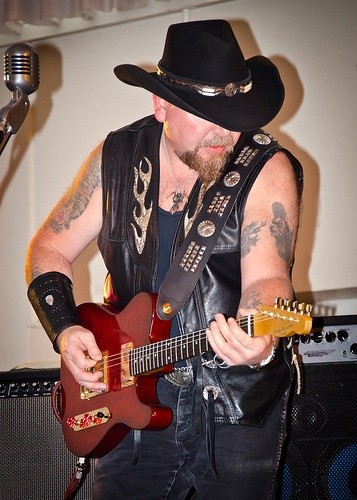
[162,131,200,214]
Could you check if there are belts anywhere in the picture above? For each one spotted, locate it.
[161,367,195,387]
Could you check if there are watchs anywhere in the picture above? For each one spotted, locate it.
[248,341,277,370]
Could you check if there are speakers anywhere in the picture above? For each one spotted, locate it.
[273,361,357,500]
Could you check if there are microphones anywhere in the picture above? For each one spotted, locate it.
[0,42,40,134]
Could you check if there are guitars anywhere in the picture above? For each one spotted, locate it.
[59,292,313,459]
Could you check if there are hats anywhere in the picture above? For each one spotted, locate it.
[113,19,285,132]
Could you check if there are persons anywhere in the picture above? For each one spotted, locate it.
[25,21,304,500]
